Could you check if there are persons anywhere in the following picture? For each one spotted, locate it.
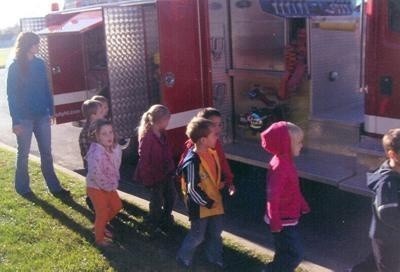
[260,121,310,272]
[6,32,71,198]
[351,128,400,272]
[79,95,236,272]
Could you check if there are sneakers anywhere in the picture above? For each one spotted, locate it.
[24,192,34,198]
[56,189,70,196]
[97,228,113,247]
[177,255,191,266]
[210,257,224,266]
